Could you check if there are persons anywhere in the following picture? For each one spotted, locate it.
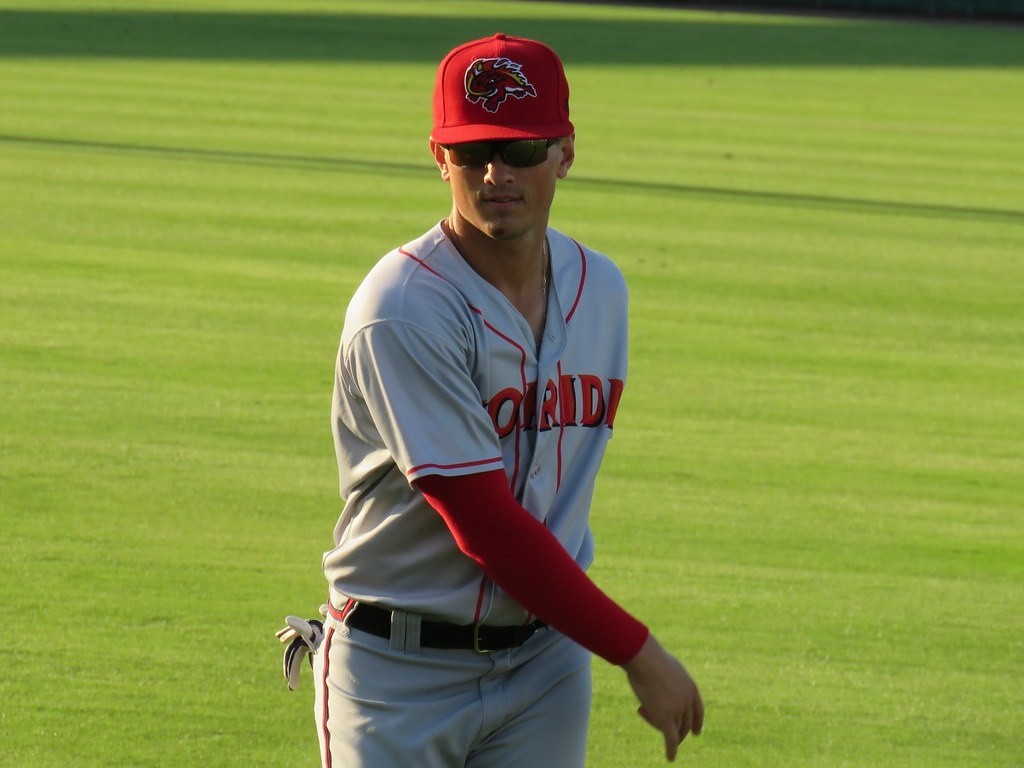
[274,27,704,768]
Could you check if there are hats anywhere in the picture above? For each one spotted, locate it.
[430,32,573,145]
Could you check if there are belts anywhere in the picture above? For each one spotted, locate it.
[325,594,546,653]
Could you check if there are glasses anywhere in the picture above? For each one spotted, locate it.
[436,138,562,167]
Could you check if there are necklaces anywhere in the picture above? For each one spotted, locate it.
[536,240,548,352]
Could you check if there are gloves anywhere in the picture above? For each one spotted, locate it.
[273,615,323,692]
